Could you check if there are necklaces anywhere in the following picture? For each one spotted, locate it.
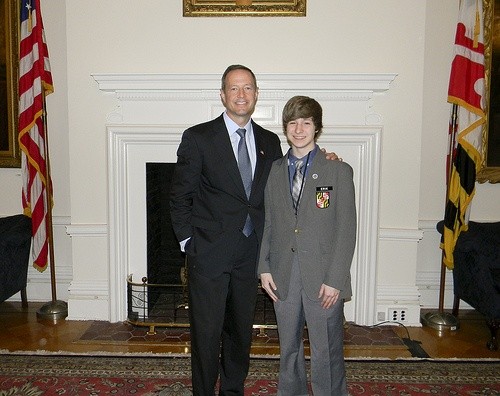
[288,148,311,216]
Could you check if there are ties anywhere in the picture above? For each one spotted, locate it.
[236,129,255,238]
[292,159,304,215]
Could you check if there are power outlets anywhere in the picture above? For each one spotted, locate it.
[388,308,408,324]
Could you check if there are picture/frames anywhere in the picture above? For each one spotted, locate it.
[0,1,20,168]
[183,0,308,18]
[476,0,500,184]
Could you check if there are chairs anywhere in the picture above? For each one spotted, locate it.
[454,219,500,352]
[0,214,32,318]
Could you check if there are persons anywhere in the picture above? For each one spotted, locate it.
[170,65,340,396]
[258,96,357,396]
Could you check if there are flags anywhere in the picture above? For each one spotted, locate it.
[17,0,54,273]
[440,0,491,270]
